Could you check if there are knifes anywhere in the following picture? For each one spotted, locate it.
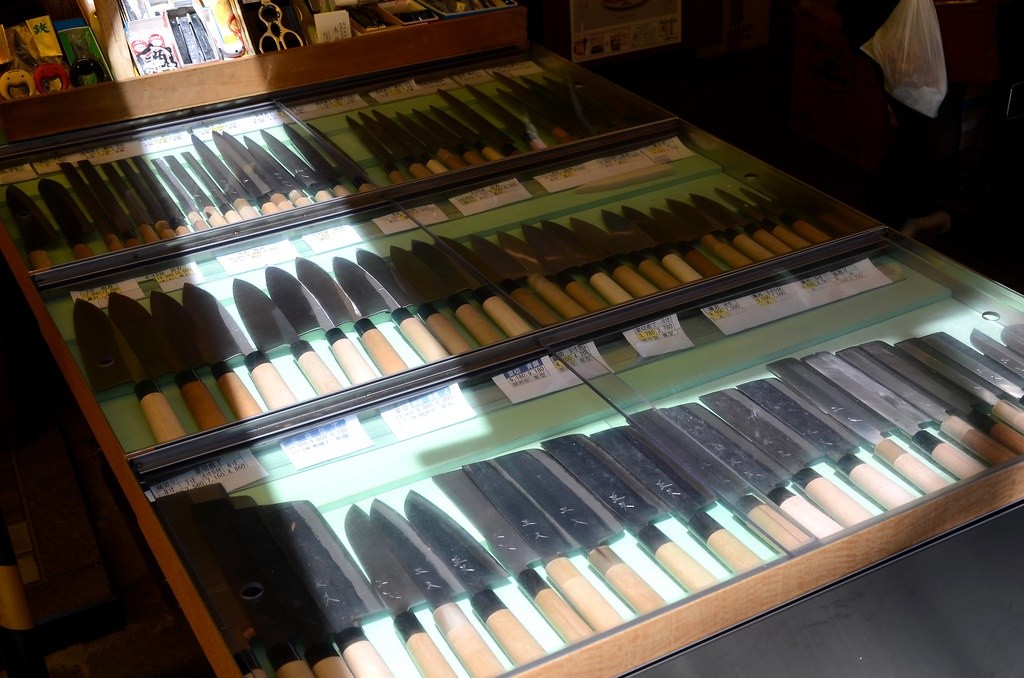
[7,72,1024,678]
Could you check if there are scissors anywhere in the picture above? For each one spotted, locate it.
[130,34,164,55]
[257,0,304,55]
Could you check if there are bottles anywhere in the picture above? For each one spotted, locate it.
[68,29,106,86]
